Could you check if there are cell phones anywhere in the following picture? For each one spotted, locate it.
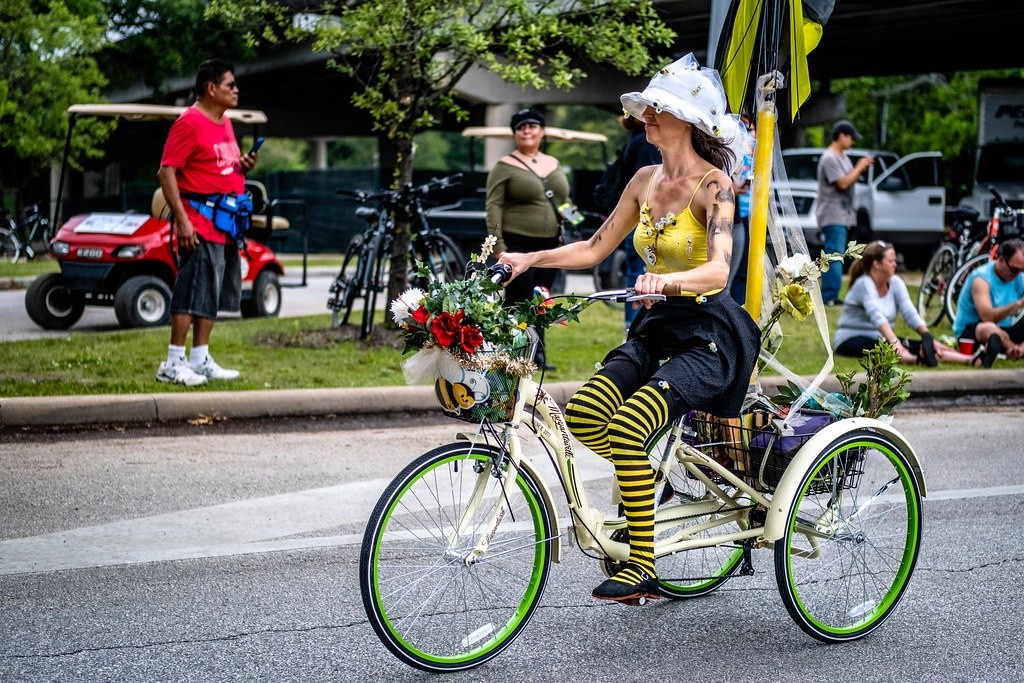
[246,137,265,162]
[558,203,585,225]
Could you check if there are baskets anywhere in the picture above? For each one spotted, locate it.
[434,341,526,423]
[687,412,864,496]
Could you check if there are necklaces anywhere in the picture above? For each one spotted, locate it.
[519,150,539,164]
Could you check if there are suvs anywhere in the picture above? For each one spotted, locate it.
[957,140,1024,223]
[765,147,949,255]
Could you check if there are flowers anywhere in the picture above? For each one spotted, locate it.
[641,211,676,267]
[623,63,722,135]
[390,221,587,353]
[751,242,872,386]
[657,292,718,390]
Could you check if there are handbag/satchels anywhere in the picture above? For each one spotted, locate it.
[189,191,253,240]
[557,201,586,245]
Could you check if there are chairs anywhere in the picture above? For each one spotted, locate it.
[150,189,171,219]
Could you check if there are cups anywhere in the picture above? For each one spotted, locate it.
[959,339,974,354]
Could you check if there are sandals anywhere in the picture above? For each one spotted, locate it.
[972,333,1002,369]
[917,332,937,367]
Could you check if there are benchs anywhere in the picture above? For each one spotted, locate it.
[244,180,305,237]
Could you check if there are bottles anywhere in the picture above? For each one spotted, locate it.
[966,242,982,262]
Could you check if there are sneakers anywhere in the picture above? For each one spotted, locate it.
[157,360,207,386]
[193,355,239,380]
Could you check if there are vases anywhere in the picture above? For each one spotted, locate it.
[701,416,756,460]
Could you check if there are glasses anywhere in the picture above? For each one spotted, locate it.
[878,241,887,250]
[1005,259,1023,274]
[218,82,236,90]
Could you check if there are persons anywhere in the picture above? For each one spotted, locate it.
[732,152,751,307]
[951,238,1024,361]
[616,113,663,341]
[816,121,876,306]
[156,58,258,387]
[486,108,578,371]
[497,52,747,600]
[832,241,1001,369]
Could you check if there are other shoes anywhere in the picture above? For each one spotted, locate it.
[824,299,841,308]
[618,471,675,518]
[535,352,557,372]
[591,564,660,601]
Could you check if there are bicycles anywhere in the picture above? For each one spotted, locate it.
[916,184,1022,331]
[327,173,473,339]
[357,282,931,673]
[0,199,56,265]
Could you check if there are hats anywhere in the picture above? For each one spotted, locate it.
[620,54,752,178]
[834,120,863,142]
[510,109,546,131]
